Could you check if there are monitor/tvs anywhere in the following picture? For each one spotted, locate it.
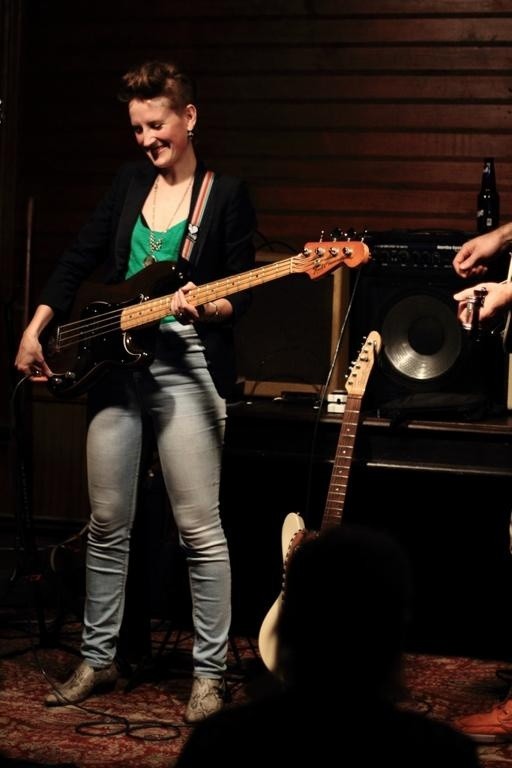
[223,242,349,403]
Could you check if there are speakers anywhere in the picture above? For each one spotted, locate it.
[348,227,512,420]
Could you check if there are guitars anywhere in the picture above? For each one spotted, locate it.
[29,229,369,402]
[258,331,382,683]
[464,242,511,353]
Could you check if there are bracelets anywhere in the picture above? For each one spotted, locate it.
[208,302,218,323]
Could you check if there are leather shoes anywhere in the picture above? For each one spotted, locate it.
[46,663,117,704]
[185,677,225,722]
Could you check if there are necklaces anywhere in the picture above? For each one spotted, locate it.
[143,173,193,268]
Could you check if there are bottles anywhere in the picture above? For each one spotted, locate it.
[475,158,500,242]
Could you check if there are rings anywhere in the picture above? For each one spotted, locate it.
[176,311,182,319]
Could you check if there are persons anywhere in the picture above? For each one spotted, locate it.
[15,60,255,724]
[174,528,481,768]
[453,220,512,682]
